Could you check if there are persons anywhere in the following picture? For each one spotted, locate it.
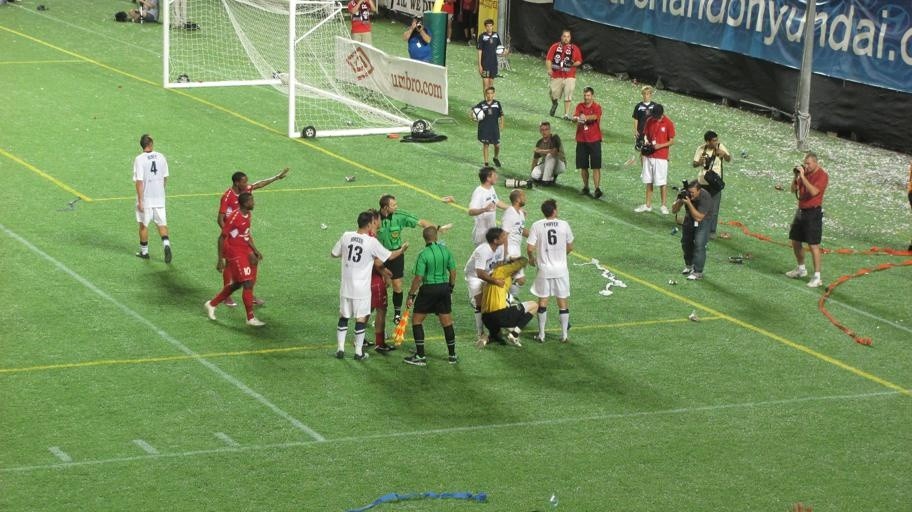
[632,104,675,214]
[632,85,657,152]
[217,167,289,307]
[784,153,828,288]
[693,130,730,241]
[203,192,265,329]
[672,181,713,281]
[331,0,603,366]
[129,0,159,23]
[133,133,172,263]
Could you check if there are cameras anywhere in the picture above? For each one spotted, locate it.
[794,165,805,173]
[642,145,653,156]
[678,179,691,199]
[704,158,711,170]
[417,16,421,30]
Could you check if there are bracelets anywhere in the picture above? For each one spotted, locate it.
[275,175,280,179]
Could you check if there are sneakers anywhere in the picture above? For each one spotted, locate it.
[163,244,173,263]
[682,263,705,281]
[493,157,502,168]
[135,251,151,260]
[541,173,559,187]
[633,203,653,214]
[578,187,604,199]
[804,276,824,288]
[203,294,268,328]
[549,103,572,120]
[659,204,670,215]
[709,231,717,241]
[334,315,569,367]
[784,266,808,278]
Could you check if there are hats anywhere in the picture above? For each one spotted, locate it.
[650,103,665,120]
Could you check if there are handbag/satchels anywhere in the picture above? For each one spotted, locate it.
[703,168,726,193]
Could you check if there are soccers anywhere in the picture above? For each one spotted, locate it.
[472,108,485,121]
[496,45,505,56]
